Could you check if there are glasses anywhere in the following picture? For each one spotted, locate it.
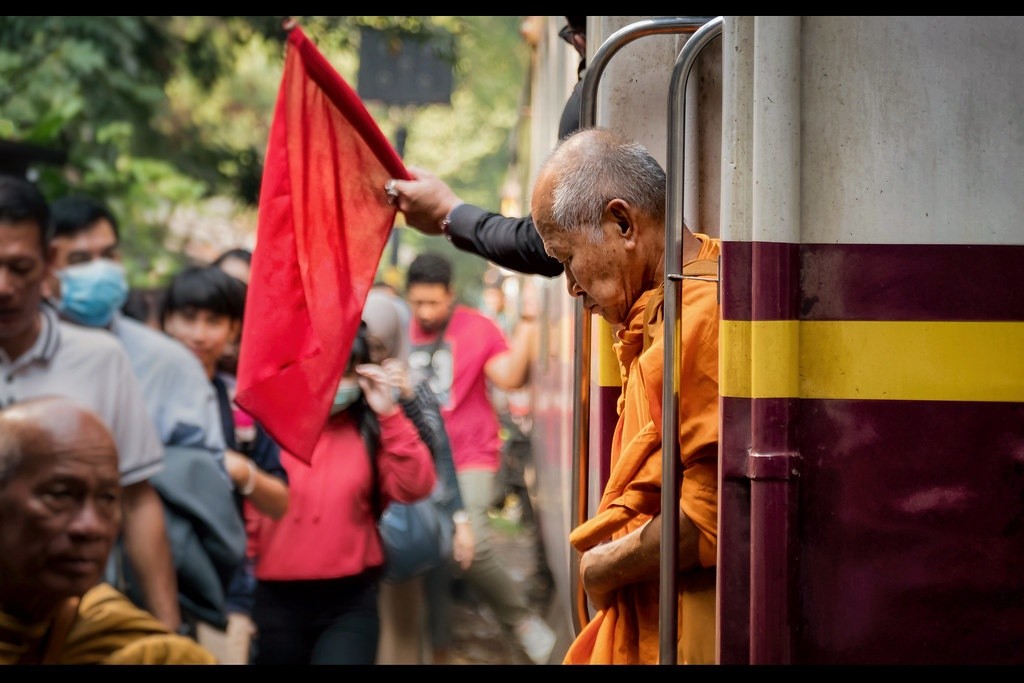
[559,26,581,46]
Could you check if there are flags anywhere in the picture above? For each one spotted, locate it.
[231,26,417,469]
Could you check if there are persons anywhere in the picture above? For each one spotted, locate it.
[247,318,438,664]
[356,285,476,662]
[208,246,257,286]
[0,171,183,631]
[400,247,558,665]
[114,287,153,328]
[1,393,223,666]
[154,263,292,665]
[529,127,720,665]
[36,184,234,591]
[383,15,590,278]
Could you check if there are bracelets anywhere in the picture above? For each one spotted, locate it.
[438,202,463,242]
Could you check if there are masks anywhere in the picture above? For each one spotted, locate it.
[45,258,128,328]
[329,386,362,416]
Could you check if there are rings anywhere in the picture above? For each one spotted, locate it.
[385,179,399,204]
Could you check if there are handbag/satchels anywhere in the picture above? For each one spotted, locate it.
[374,500,452,580]
